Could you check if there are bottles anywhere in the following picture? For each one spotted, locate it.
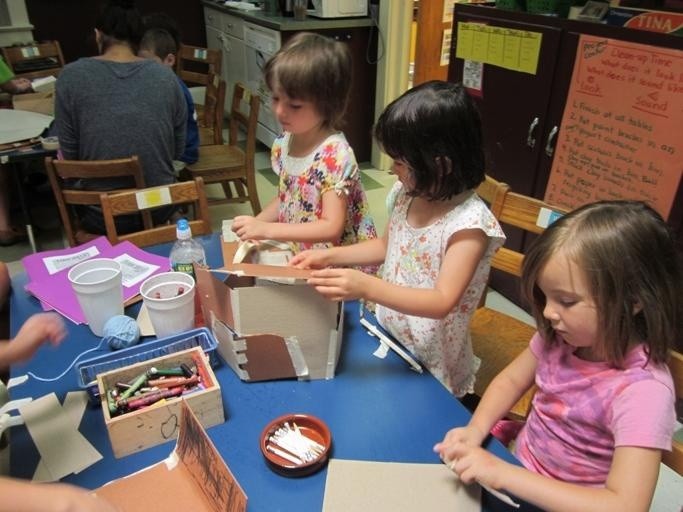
[170,220,207,281]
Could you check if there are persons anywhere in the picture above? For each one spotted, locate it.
[229,32,380,314]
[0,311,113,512]
[0,262,11,304]
[54,0,190,237]
[0,54,33,96]
[0,160,49,246]
[433,199,683,512]
[285,80,506,402]
[136,28,201,177]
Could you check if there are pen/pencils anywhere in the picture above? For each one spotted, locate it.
[105,362,205,414]
[266,420,326,465]
[156,292,160,299]
[175,287,185,297]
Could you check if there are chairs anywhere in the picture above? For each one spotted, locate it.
[172,43,224,84]
[4,40,66,88]
[184,83,261,217]
[100,178,214,248]
[44,153,150,246]
[179,75,233,198]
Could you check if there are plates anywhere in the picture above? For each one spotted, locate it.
[261,413,332,478]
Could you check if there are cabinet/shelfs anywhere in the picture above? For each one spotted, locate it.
[446,4,683,424]
[203,0,378,168]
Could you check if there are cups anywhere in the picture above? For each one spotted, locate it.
[139,273,197,338]
[293,0,308,21]
[67,259,125,337]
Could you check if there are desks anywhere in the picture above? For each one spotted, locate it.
[0,228,534,512]
[0,86,91,254]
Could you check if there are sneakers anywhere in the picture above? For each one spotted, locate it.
[0,224,40,246]
